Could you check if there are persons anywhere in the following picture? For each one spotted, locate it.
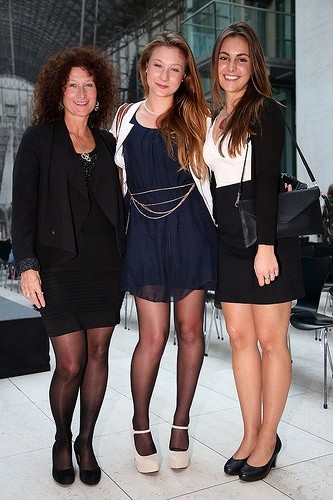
[12,48,126,486]
[204,22,299,480]
[108,31,217,475]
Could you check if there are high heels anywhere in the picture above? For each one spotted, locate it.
[132,429,162,473]
[168,426,191,469]
[240,434,282,481]
[74,436,101,485]
[52,441,75,484]
[225,457,248,474]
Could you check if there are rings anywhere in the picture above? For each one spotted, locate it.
[37,292,43,294]
[265,277,270,280]
[26,293,31,297]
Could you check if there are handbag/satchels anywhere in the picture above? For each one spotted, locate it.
[239,185,326,249]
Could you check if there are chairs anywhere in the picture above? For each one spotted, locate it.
[0,237,333,410]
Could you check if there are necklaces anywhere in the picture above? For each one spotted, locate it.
[144,98,168,115]
[69,131,92,162]
